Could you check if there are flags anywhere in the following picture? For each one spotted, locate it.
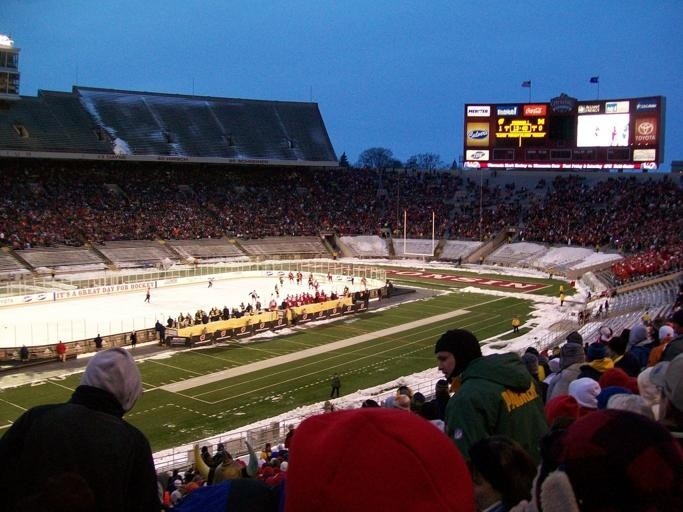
[590,76,600,83]
[520,80,531,88]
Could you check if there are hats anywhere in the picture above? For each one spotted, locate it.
[435,329,483,383]
[285,408,474,511]
[521,326,683,416]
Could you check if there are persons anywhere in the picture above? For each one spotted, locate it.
[0,156,683,512]
